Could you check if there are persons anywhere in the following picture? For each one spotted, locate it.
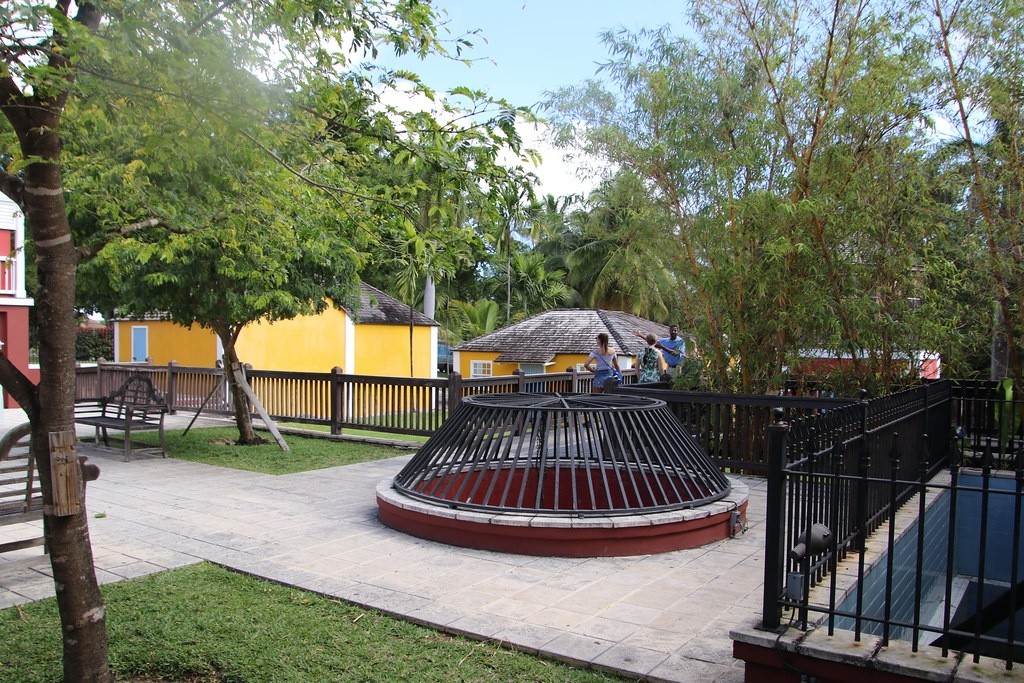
[635,333,663,384]
[582,333,621,427]
[634,325,685,377]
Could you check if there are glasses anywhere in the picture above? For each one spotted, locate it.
[595,337,598,339]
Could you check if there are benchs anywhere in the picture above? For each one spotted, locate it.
[74,375,169,463]
[0,423,101,555]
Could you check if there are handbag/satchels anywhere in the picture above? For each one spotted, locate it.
[612,369,622,383]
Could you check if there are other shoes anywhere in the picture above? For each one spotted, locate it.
[583,421,592,427]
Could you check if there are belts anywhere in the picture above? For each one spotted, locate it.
[668,365,678,369]
